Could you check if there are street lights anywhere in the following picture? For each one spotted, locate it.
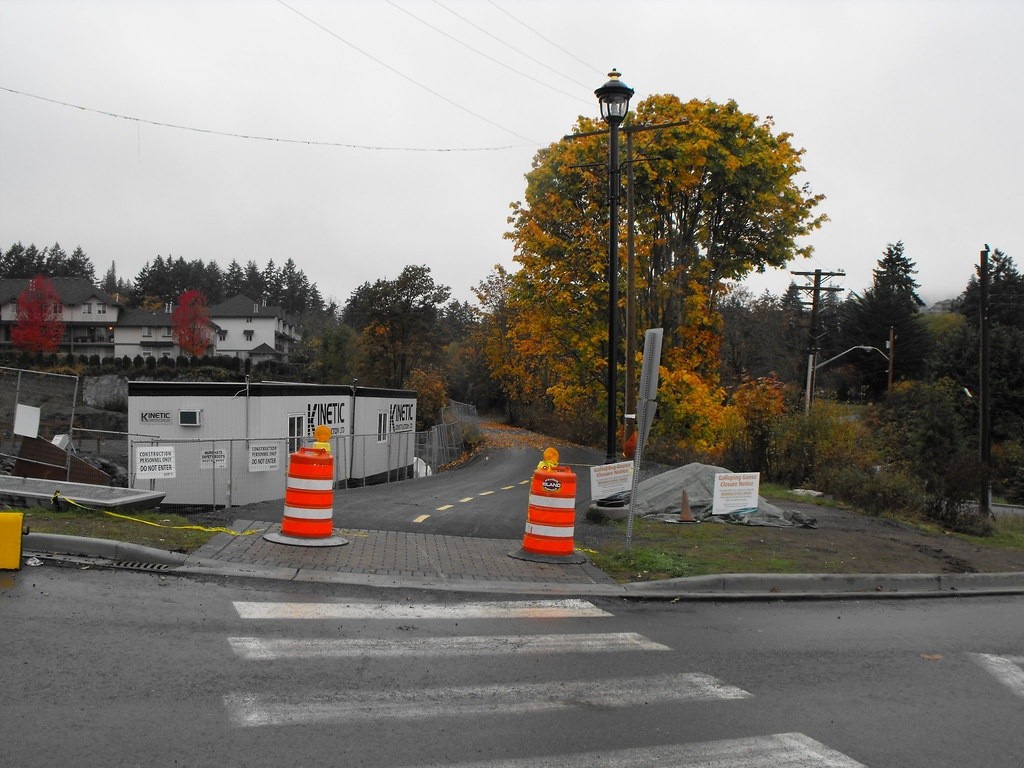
[583,69,638,528]
[805,346,873,414]
[866,345,894,394]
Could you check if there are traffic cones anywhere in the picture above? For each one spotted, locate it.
[678,490,699,523]
